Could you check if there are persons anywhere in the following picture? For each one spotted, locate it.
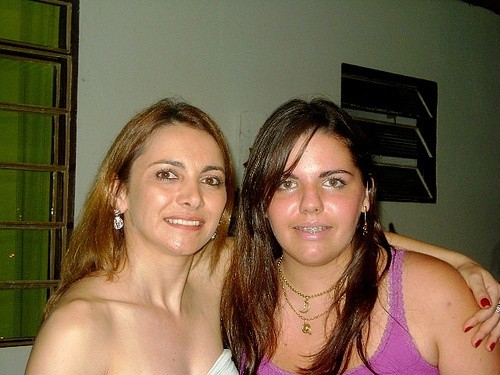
[277,255,348,333]
[220,98,499,375]
[25,98,500,375]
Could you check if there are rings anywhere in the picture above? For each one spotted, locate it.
[495,304,500,312]
